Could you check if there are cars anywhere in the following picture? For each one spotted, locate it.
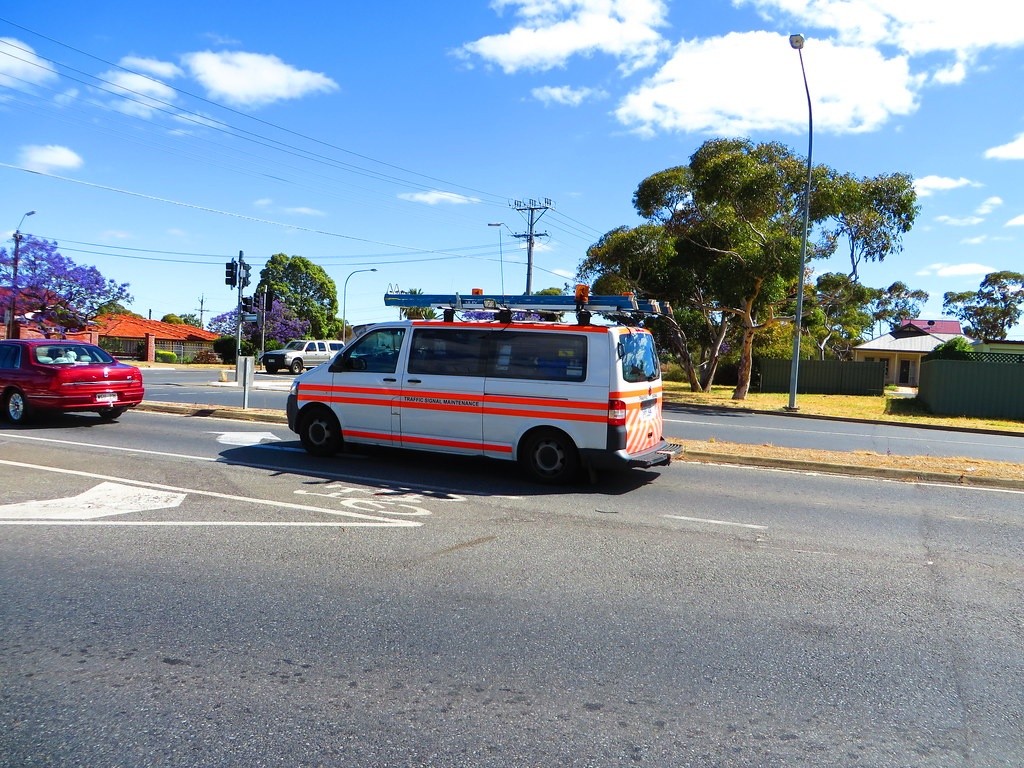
[0,336,145,426]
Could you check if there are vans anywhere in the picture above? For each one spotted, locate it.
[286,305,684,485]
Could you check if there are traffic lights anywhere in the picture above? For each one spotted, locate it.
[254,292,260,307]
[225,261,237,287]
[243,263,252,287]
[243,296,253,313]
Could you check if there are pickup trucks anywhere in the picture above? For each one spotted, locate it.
[262,339,347,375]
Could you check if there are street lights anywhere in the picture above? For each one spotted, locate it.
[343,268,378,343]
[783,34,814,412]
[488,223,505,304]
[9,210,37,339]
